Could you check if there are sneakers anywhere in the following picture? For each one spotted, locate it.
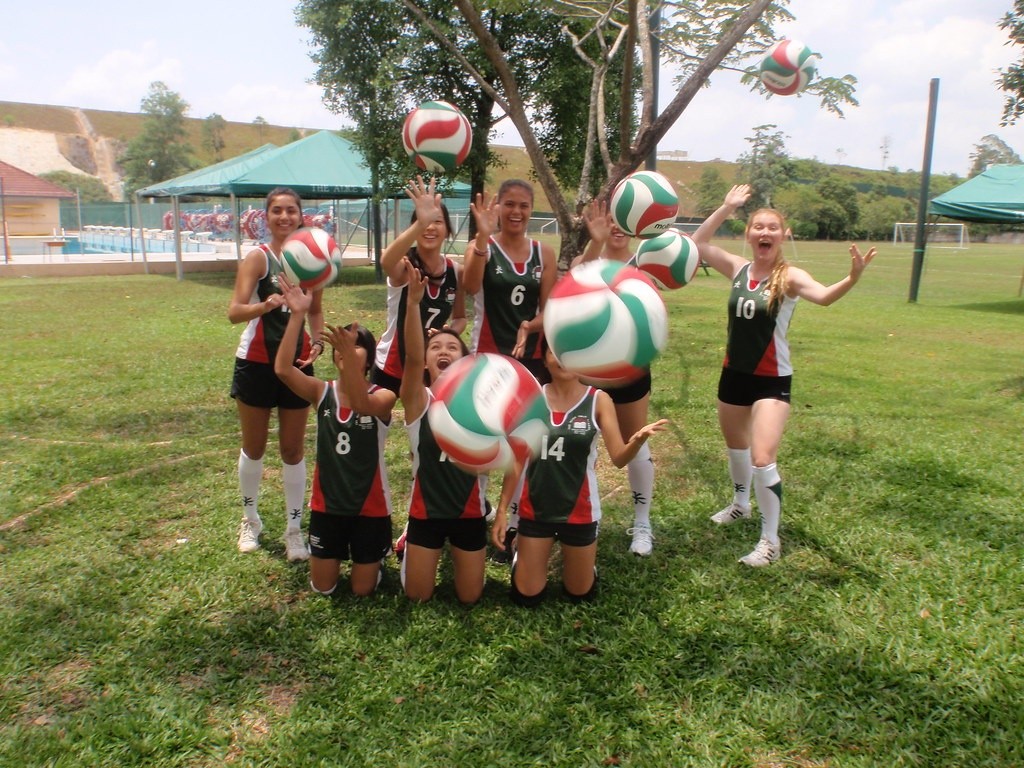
[490,528,518,566]
[738,536,781,567]
[238,511,262,553]
[283,526,311,564]
[710,501,751,526]
[625,520,654,559]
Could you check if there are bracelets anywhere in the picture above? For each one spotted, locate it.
[473,243,489,256]
[312,341,324,355]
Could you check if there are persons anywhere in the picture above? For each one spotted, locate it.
[464,179,557,386]
[394,257,487,604]
[491,336,668,607]
[371,176,463,395]
[694,184,878,568]
[571,198,654,558]
[275,272,396,597]
[229,186,325,561]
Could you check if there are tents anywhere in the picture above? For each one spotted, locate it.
[175,130,473,278]
[134,143,398,274]
[925,163,1024,296]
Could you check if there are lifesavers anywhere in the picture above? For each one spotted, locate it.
[163,210,339,243]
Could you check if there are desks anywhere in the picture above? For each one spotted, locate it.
[42,241,70,262]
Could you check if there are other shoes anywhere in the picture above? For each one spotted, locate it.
[397,520,410,553]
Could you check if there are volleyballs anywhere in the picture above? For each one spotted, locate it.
[609,169,680,241]
[634,226,701,292]
[401,99,475,174]
[426,350,551,477]
[542,258,672,390]
[278,225,344,294]
[759,39,816,97]
